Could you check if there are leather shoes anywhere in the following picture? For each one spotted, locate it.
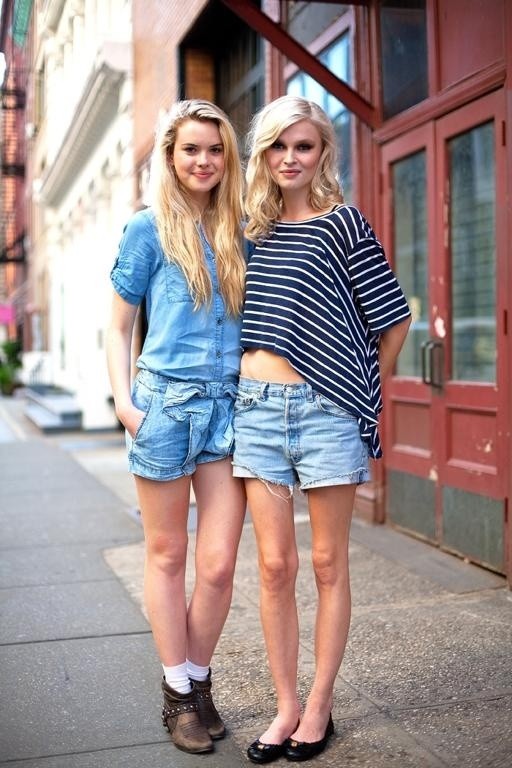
[287,714,333,759]
[247,713,299,764]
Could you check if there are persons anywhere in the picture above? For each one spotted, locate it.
[103,99,259,756]
[227,93,414,763]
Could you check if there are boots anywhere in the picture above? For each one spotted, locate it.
[160,668,226,754]
